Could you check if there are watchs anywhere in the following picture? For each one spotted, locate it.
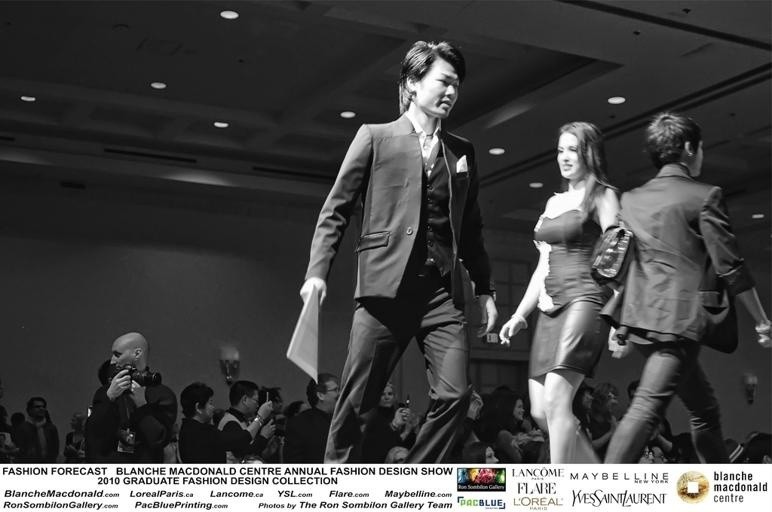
[481,289,497,301]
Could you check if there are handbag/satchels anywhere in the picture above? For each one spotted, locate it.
[589,227,631,286]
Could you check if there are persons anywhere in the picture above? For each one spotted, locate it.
[601,112,772,462]
[299,41,498,463]
[0,331,772,463]
[499,121,619,462]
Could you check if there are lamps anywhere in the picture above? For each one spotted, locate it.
[743,372,759,404]
[219,342,240,385]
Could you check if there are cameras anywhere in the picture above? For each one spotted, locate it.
[124,364,161,385]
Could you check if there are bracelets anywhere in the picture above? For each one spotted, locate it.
[754,321,772,334]
[509,312,528,329]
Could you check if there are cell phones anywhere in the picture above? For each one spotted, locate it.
[266,392,269,402]
